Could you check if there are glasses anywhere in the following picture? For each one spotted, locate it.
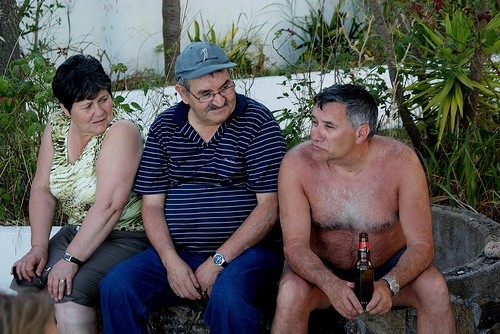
[187,82,236,103]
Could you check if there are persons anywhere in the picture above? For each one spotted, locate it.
[270,84,458,334]
[100,41,286,334]
[9,54,149,334]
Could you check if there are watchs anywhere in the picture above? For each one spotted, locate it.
[210,251,230,269]
[63,252,84,265]
[382,275,399,296]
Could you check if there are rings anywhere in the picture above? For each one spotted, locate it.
[59,280,65,288]
[201,291,206,296]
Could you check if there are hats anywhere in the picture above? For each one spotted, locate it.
[174,42,237,80]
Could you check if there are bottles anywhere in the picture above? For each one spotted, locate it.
[354,232,375,310]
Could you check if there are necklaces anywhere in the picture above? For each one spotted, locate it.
[78,142,83,156]
